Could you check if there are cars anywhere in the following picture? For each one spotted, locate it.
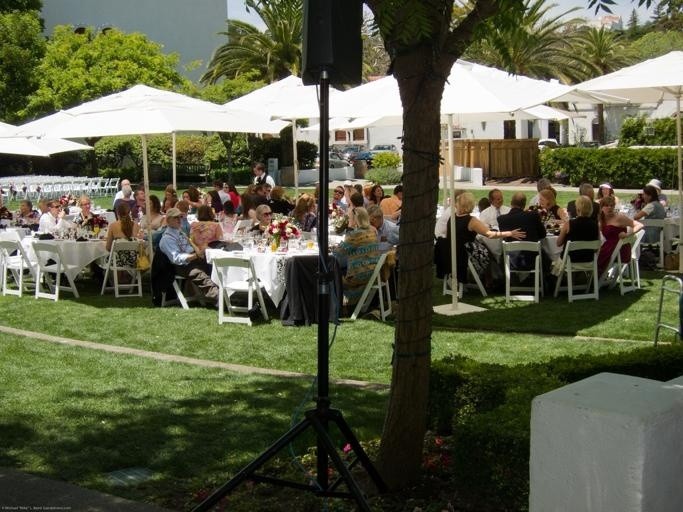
[316,151,350,168]
[537,138,559,149]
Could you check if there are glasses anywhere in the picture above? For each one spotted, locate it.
[225,187,231,188]
[334,189,344,194]
[51,204,62,209]
[264,211,273,216]
[175,215,184,219]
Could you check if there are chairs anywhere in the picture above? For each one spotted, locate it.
[100,241,145,298]
[502,241,544,304]
[598,236,637,295]
[150,231,206,309]
[553,241,601,303]
[638,221,665,271]
[0,178,108,198]
[1,241,44,299]
[32,242,81,300]
[351,251,395,322]
[231,221,251,236]
[95,179,109,196]
[211,257,269,326]
[436,238,487,299]
[106,178,120,198]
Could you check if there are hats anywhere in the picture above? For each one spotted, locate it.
[599,182,613,189]
[645,179,662,190]
[165,207,185,217]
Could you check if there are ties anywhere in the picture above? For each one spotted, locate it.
[178,229,205,260]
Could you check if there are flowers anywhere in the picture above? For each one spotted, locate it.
[261,214,301,250]
[88,215,109,231]
[59,194,74,208]
[333,207,346,225]
[527,203,549,230]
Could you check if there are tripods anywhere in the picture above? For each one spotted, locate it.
[195,70,386,512]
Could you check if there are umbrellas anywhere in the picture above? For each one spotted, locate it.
[571,49,682,275]
[1,122,93,158]
[227,75,356,192]
[300,106,569,210]
[329,57,575,316]
[9,83,291,195]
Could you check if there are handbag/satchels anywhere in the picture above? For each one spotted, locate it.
[136,242,151,271]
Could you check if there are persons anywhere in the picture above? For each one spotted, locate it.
[435,189,480,239]
[369,184,384,207]
[380,185,402,215]
[596,183,619,205]
[113,179,133,208]
[218,200,238,234]
[253,185,268,207]
[36,185,42,203]
[288,194,316,233]
[497,192,551,273]
[634,186,666,243]
[182,190,202,214]
[159,208,219,309]
[16,200,40,225]
[213,181,231,205]
[143,195,165,230]
[391,174,404,225]
[248,204,272,235]
[270,186,295,217]
[529,178,552,205]
[351,184,368,209]
[368,206,400,245]
[0,184,5,209]
[579,183,595,199]
[21,183,28,199]
[189,206,223,252]
[332,207,381,315]
[556,196,600,264]
[132,190,146,221]
[536,189,568,222]
[238,193,256,220]
[253,163,275,199]
[224,183,240,208]
[243,184,256,194]
[38,200,71,238]
[597,196,643,276]
[447,192,527,291]
[105,199,139,284]
[162,188,178,213]
[206,190,222,213]
[332,185,350,210]
[334,193,364,233]
[480,189,512,227]
[263,183,271,200]
[646,178,667,206]
[72,196,100,227]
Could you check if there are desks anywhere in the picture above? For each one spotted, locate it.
[22,230,107,285]
[621,208,679,253]
[205,235,345,308]
[475,224,605,282]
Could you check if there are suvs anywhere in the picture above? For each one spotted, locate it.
[370,143,397,154]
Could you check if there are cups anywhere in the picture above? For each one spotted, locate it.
[308,239,313,249]
[94,224,100,240]
[271,240,277,252]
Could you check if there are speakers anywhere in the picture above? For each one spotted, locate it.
[301,1,363,87]
